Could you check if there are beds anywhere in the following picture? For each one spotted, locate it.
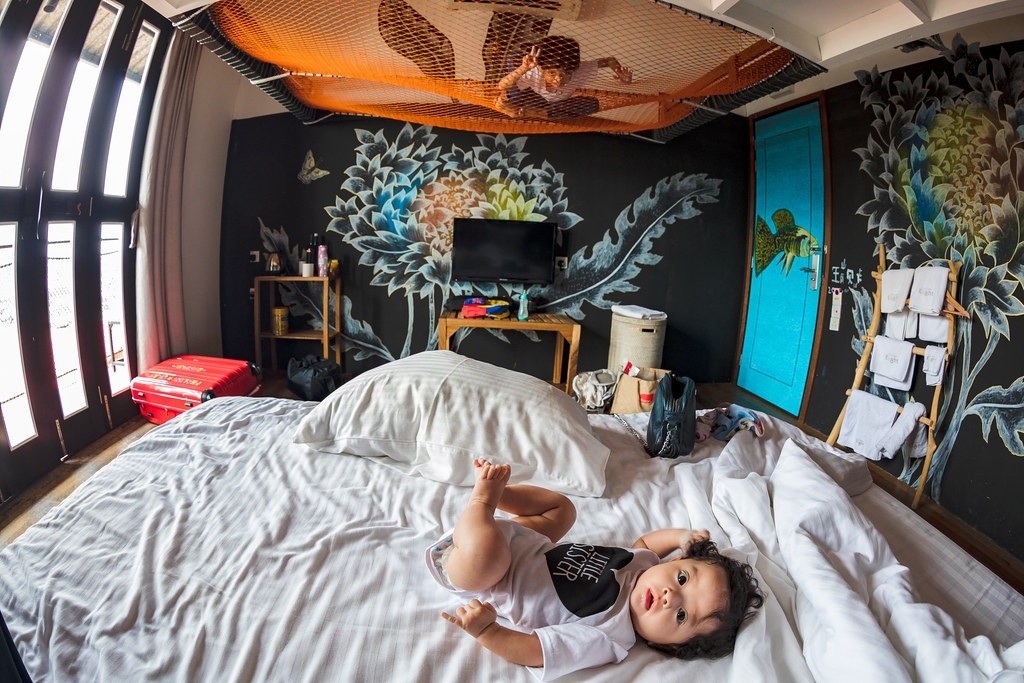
[0,394,1024,683]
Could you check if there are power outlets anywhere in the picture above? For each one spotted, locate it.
[249,250,259,263]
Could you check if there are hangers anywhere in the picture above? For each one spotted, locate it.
[906,290,971,319]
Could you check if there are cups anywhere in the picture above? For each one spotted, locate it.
[302,264,314,277]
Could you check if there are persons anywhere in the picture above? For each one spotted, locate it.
[427,458,768,682]
[495,34,633,120]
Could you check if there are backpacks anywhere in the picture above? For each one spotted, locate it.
[462,296,511,319]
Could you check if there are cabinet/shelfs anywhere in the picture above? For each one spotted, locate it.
[253,276,341,384]
[438,311,581,396]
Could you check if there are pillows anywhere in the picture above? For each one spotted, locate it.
[291,349,611,499]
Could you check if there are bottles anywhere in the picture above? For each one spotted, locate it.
[318,236,328,277]
[305,233,316,276]
[517,290,529,321]
[312,233,319,275]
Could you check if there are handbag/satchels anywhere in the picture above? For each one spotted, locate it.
[285,355,342,401]
[646,372,697,459]
[609,368,672,415]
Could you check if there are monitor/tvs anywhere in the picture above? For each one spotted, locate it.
[451,218,559,285]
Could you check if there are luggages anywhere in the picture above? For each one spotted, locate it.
[130,354,262,426]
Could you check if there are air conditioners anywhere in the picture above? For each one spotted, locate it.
[445,0,583,21]
